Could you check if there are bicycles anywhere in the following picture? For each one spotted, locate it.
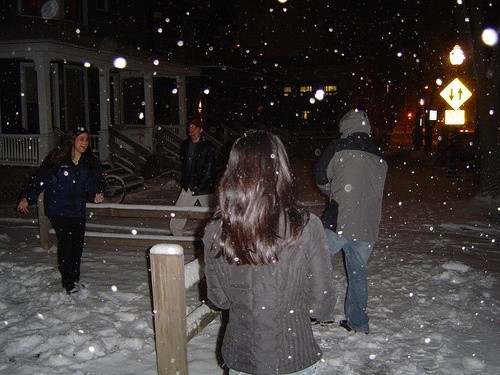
[100,164,126,203]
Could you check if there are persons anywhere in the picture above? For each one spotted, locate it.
[17,124,110,294]
[169,119,217,237]
[202,129,337,375]
[310,106,389,335]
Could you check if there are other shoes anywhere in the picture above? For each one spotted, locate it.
[62,279,85,288]
[339,320,369,335]
[66,281,78,294]
[309,317,335,325]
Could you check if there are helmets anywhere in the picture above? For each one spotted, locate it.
[337,109,371,139]
